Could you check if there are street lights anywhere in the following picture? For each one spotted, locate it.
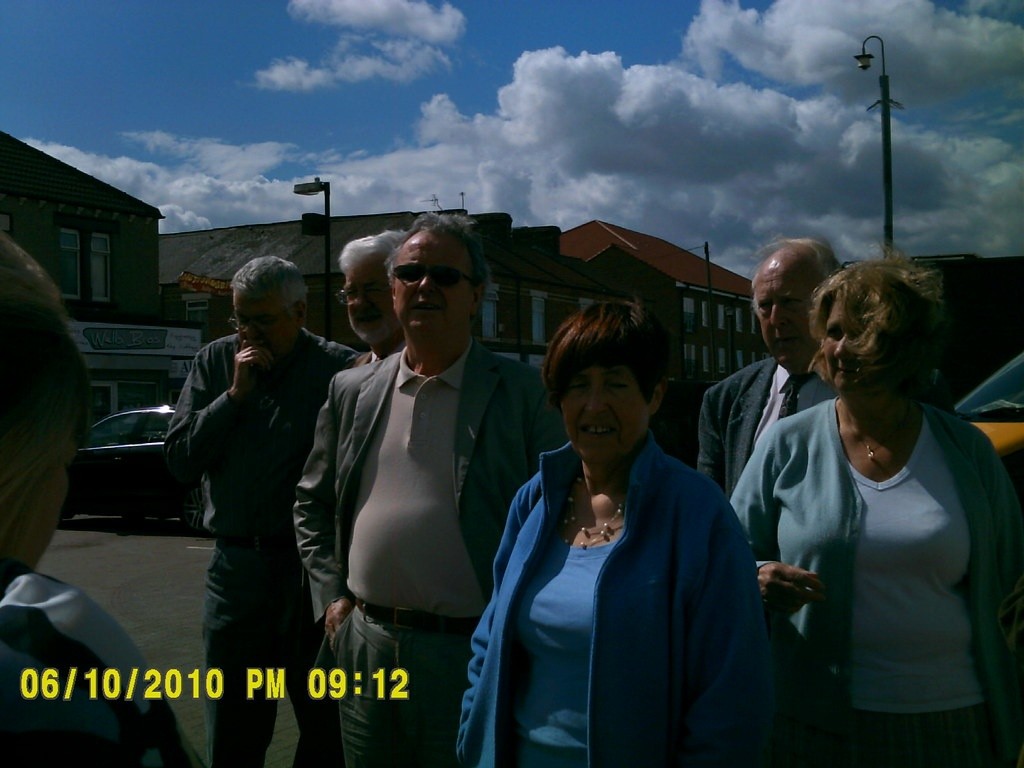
[853,32,901,257]
[293,180,334,342]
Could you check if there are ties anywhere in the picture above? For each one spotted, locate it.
[777,374,813,418]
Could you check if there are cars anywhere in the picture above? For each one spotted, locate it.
[59,402,211,539]
[946,353,1023,488]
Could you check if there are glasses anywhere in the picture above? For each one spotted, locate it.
[393,264,475,287]
[335,285,391,304]
[228,309,290,331]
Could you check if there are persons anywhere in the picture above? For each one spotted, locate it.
[0,231,197,768]
[729,259,1024,768]
[165,257,363,768]
[293,213,570,768]
[696,239,844,500]
[339,229,411,368]
[456,299,767,768]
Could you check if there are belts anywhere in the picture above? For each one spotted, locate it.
[222,536,293,552]
[356,598,481,637]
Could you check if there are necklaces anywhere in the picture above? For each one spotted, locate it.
[562,478,626,548]
[866,403,911,458]
